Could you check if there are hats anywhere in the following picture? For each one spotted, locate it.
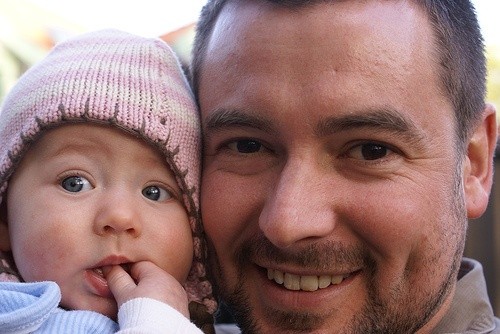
[0,30,217,326]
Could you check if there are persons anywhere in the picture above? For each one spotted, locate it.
[190,0,500,334]
[0,28,220,334]
